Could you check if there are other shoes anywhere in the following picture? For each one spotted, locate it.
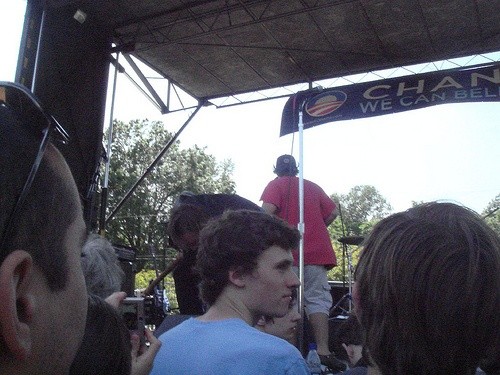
[318,352,347,371]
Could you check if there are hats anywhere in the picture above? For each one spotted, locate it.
[273,154,296,172]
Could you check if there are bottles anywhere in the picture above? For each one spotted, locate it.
[307,343,321,375]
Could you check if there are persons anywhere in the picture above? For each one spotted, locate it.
[259,154,347,372]
[75,181,313,375]
[0,106,87,375]
[333,201,500,375]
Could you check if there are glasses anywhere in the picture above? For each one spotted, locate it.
[0,81,71,267]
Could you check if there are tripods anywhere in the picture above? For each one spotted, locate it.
[329,245,353,320]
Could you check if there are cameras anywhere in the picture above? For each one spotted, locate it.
[118,297,145,351]
